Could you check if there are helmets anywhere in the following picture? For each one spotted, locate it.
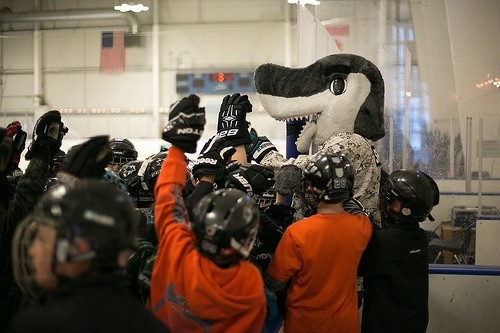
[106,138,166,209]
[14,179,126,294]
[301,152,354,217]
[192,188,260,270]
[378,170,436,224]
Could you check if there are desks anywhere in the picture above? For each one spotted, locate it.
[442,220,476,265]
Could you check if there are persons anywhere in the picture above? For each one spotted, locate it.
[150,93,267,333]
[29,173,171,333]
[0,93,440,333]
[264,150,372,333]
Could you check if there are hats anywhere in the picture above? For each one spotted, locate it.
[275,164,302,195]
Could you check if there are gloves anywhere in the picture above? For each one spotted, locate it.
[25,110,64,160]
[162,94,206,153]
[248,131,276,161]
[0,121,28,176]
[191,135,240,180]
[227,161,276,193]
[217,94,252,147]
[61,135,113,182]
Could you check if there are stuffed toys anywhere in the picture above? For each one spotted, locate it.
[246,53,386,308]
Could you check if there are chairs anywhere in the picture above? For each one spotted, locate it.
[427,224,472,264]
